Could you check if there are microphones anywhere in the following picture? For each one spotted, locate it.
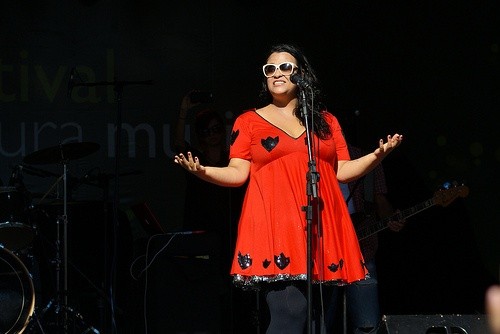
[68,173,88,191]
[289,74,311,90]
[69,72,75,88]
[164,230,208,235]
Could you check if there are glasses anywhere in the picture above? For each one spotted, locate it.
[263,62,298,78]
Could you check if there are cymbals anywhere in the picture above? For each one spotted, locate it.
[22,141,100,165]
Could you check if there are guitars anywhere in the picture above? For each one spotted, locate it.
[356,182,471,239]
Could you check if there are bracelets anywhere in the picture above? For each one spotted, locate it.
[178,117,185,121]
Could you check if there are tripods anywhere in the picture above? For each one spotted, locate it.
[28,159,109,334]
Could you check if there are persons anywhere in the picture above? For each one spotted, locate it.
[174,89,238,256]
[314,100,406,334]
[175,43,402,334]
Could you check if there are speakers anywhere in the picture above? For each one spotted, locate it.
[381,313,490,334]
[147,256,269,334]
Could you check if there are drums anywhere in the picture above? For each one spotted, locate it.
[0,243,38,334]
[0,184,42,239]
